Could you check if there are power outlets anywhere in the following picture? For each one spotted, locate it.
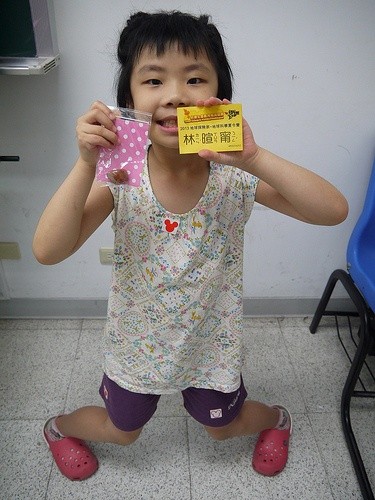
[99,247,114,265]
[0,242,21,259]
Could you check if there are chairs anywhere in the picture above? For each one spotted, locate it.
[310,158,375,500]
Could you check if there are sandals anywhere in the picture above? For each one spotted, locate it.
[42,416,98,481]
[253,404,293,477]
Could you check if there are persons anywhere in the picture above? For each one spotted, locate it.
[31,10,350,482]
